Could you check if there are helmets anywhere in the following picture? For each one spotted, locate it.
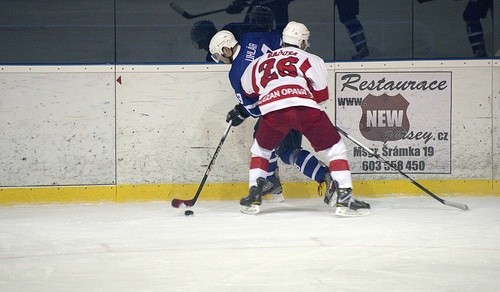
[282,21,310,50]
[189,20,216,49]
[208,31,238,63]
[248,6,276,30]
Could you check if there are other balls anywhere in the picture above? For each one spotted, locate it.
[185,210,194,216]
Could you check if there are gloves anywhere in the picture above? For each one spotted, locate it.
[225,0,248,14]
[225,103,250,126]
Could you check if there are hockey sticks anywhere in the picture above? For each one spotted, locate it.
[171,2,231,20]
[171,119,233,207]
[332,125,468,211]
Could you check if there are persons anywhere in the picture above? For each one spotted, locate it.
[209,30,338,207]
[191,19,264,62]
[225,0,293,31]
[463,0,492,58]
[249,6,276,33]
[240,20,371,217]
[335,1,370,60]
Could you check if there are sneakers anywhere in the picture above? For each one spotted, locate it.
[240,177,266,213]
[262,165,285,202]
[336,187,371,216]
[317,170,338,207]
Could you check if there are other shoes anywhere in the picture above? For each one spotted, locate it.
[352,52,369,60]
